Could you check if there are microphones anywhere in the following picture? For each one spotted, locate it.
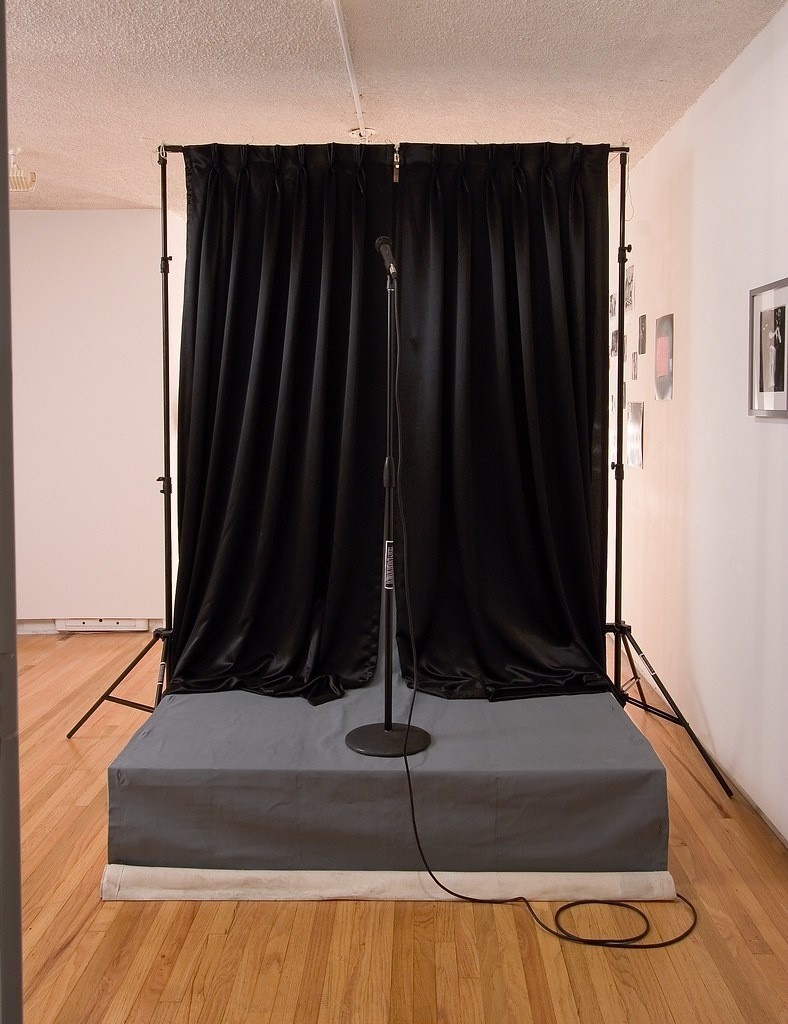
[374,235,398,279]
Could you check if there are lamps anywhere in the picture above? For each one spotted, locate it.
[9,146,37,194]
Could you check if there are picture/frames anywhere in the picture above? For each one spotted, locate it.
[748,277,788,419]
